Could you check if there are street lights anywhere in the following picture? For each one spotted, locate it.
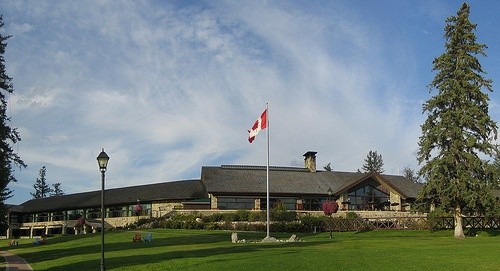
[97,147,110,271]
[137,198,140,221]
[327,188,333,239]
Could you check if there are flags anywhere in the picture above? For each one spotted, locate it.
[247,108,268,143]
